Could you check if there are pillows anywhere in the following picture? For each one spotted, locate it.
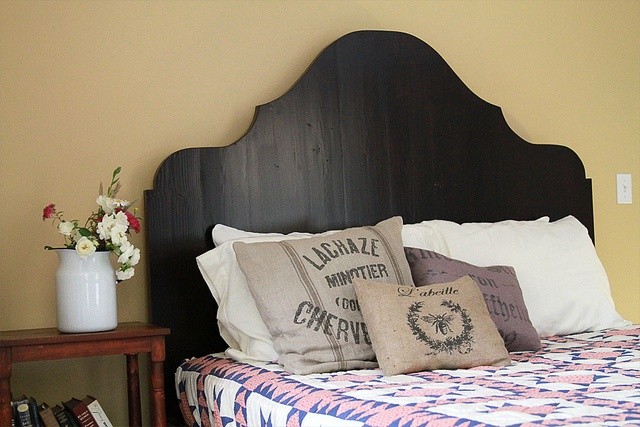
[404,246,543,353]
[232,216,415,377]
[415,212,635,338]
[351,272,514,380]
[193,219,453,367]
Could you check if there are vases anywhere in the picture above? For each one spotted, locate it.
[53,246,119,334]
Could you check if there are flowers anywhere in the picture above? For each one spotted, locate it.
[42,165,144,284]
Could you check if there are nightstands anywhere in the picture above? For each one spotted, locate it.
[0,320,172,427]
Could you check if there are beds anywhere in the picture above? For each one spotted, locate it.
[140,29,640,427]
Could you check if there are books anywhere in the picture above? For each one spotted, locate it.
[61,397,99,426]
[27,396,41,426]
[50,404,72,426]
[81,394,113,426]
[63,408,79,426]
[14,394,33,426]
[10,401,16,426]
[37,402,60,426]
